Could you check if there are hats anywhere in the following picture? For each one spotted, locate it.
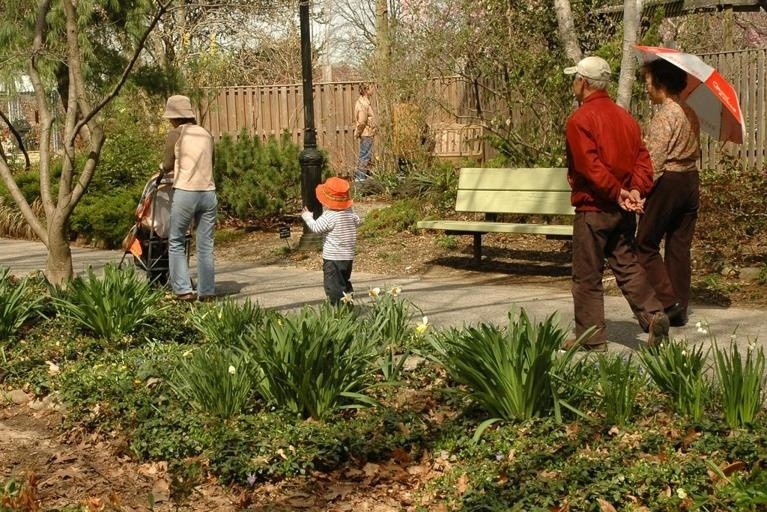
[563,56,612,82]
[162,95,196,120]
[315,177,352,210]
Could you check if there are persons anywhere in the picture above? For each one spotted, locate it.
[395,87,429,148]
[156,95,220,301]
[560,54,670,353]
[353,80,377,178]
[632,60,700,330]
[297,173,364,307]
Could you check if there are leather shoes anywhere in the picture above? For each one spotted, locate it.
[663,302,685,318]
[669,313,687,327]
[178,293,193,301]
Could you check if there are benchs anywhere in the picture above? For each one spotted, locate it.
[413,165,579,271]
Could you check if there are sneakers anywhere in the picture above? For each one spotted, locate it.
[646,311,669,354]
[560,338,607,351]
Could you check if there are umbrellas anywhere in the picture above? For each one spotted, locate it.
[628,44,748,145]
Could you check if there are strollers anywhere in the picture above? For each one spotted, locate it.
[113,163,194,293]
